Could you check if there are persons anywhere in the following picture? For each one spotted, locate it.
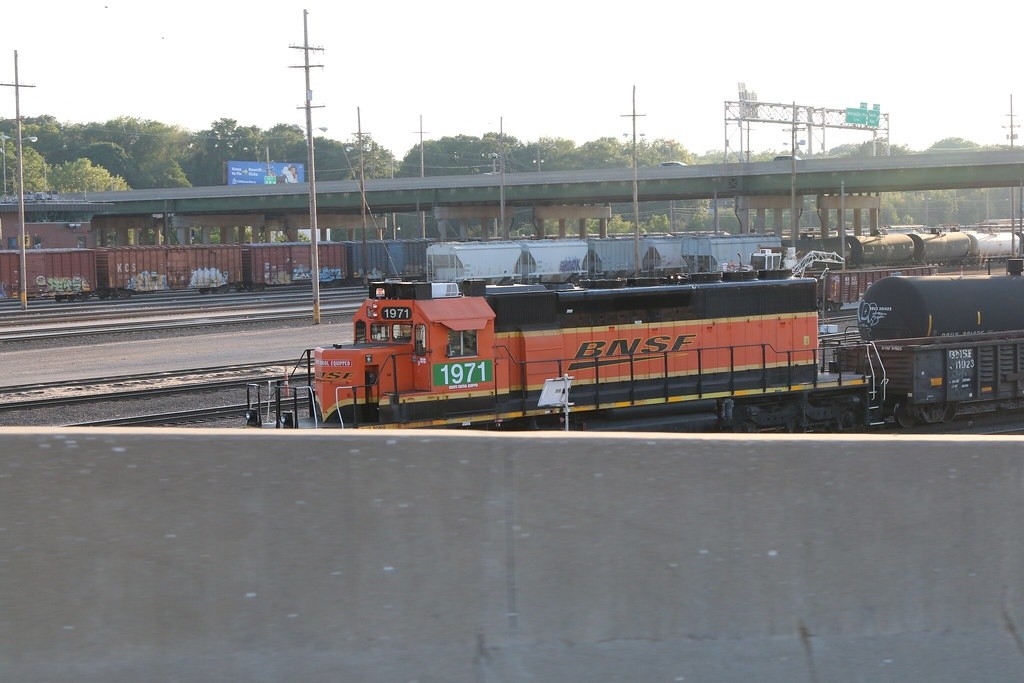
[271,163,299,183]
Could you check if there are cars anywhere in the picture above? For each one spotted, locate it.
[658,160,688,166]
[770,154,801,161]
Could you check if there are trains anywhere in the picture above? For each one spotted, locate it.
[0,223,1024,303]
[238,258,1024,433]
